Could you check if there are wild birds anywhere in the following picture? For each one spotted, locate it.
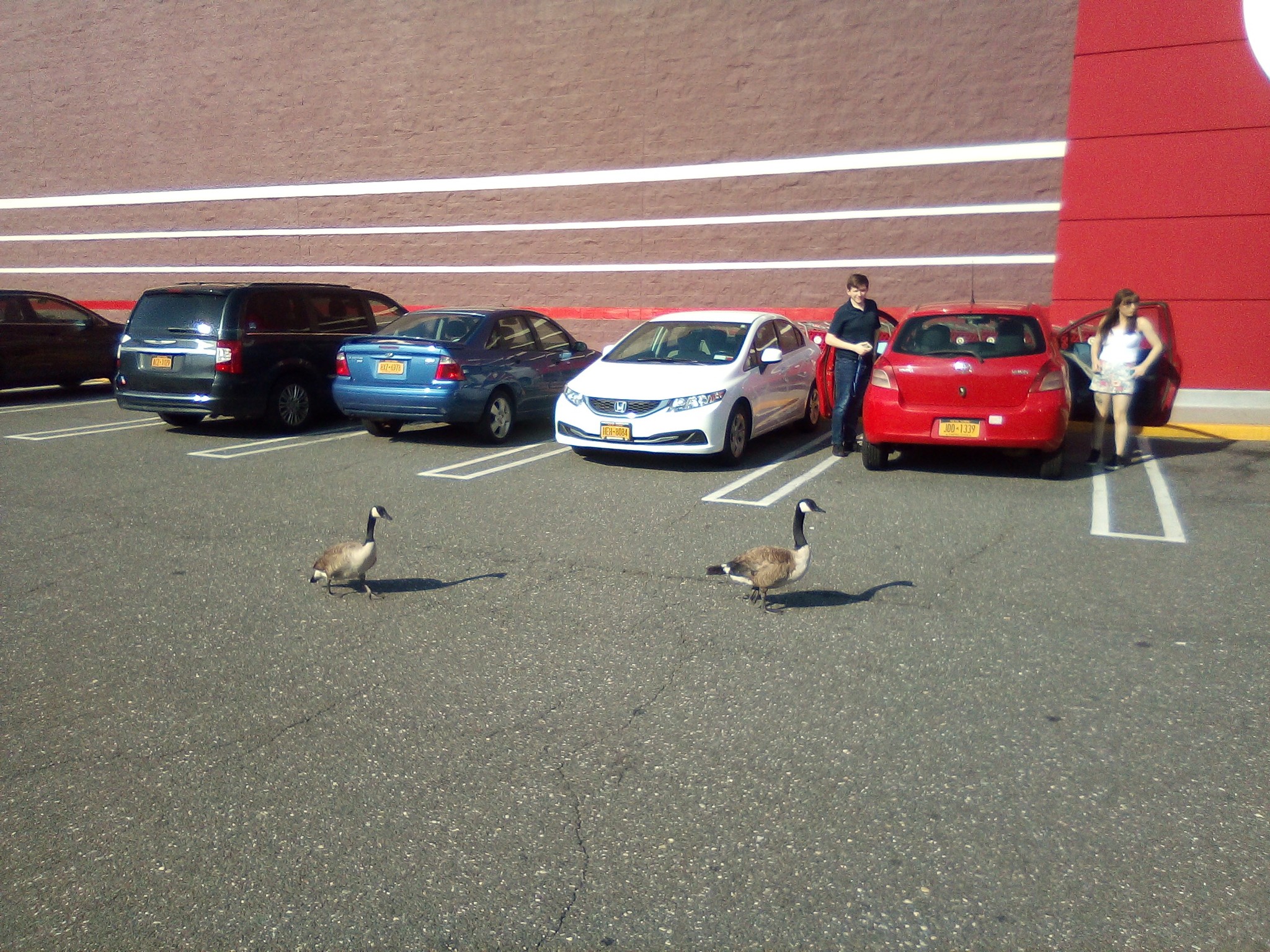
[306,502,394,602]
[702,496,828,617]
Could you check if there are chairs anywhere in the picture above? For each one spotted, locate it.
[495,326,527,351]
[922,325,950,351]
[995,321,1024,352]
[678,327,773,366]
[448,319,467,337]
[323,300,353,331]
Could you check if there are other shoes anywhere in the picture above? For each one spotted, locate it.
[1086,447,1100,466]
[844,443,863,452]
[832,445,848,456]
[1105,455,1124,471]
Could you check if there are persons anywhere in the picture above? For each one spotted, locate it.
[825,273,881,456]
[1084,287,1164,470]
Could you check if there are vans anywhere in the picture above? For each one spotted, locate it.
[113,281,433,436]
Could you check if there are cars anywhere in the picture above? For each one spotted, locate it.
[814,301,1183,481]
[0,288,126,394]
[553,308,822,466]
[332,305,604,447]
